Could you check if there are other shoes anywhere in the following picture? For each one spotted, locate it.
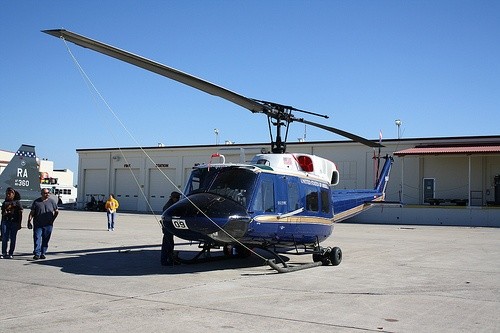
[0,253,8,258]
[40,255,46,259]
[33,255,39,260]
[160,260,174,266]
[9,253,13,258]
[112,228,114,231]
[108,229,111,231]
[168,259,180,265]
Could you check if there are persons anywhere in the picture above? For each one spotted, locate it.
[105,194,119,231]
[28,188,58,260]
[161,192,182,266]
[0,187,23,259]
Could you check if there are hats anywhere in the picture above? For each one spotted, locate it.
[171,192,182,198]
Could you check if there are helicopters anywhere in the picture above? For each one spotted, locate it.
[39,27,392,274]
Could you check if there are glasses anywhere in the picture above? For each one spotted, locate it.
[42,192,49,194]
